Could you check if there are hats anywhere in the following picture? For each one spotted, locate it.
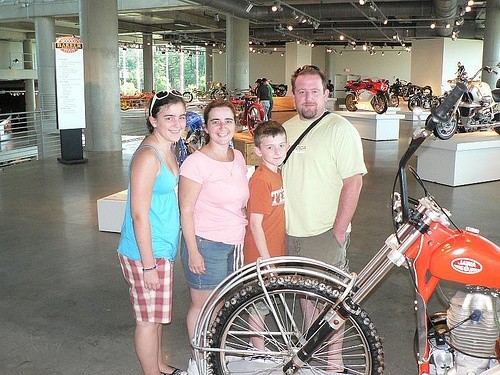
[255,79,261,83]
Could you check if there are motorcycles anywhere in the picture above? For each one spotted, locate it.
[230,95,276,140]
[187,84,500,372]
[118,61,499,144]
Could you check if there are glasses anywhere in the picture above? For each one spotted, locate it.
[296,66,319,73]
[150,89,183,116]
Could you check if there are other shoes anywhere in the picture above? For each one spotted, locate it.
[160,365,187,375]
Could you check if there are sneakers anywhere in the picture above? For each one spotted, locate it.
[244,344,277,363]
[188,358,213,375]
